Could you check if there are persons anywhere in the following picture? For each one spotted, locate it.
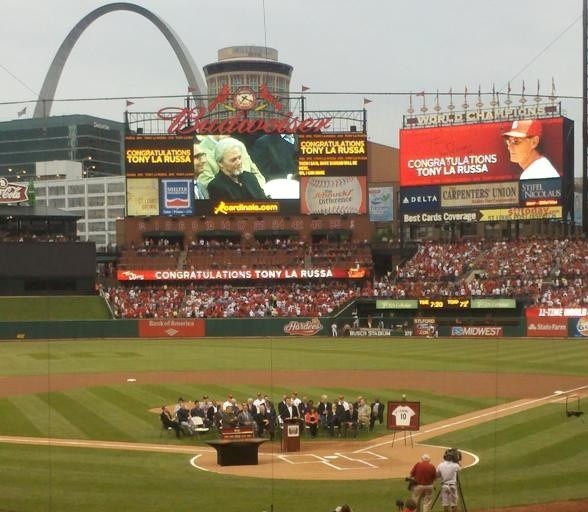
[409,452,437,512]
[402,499,416,511]
[499,121,560,178]
[367,315,373,328]
[160,385,386,438]
[435,452,465,512]
[190,135,300,201]
[331,322,338,336]
[89,233,588,325]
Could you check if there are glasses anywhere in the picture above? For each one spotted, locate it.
[194,152,206,160]
[503,138,523,146]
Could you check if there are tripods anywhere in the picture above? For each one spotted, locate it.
[430,464,467,512]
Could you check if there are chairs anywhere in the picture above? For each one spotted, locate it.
[189,416,209,441]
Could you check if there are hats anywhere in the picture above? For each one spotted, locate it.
[503,120,543,138]
[422,454,430,461]
